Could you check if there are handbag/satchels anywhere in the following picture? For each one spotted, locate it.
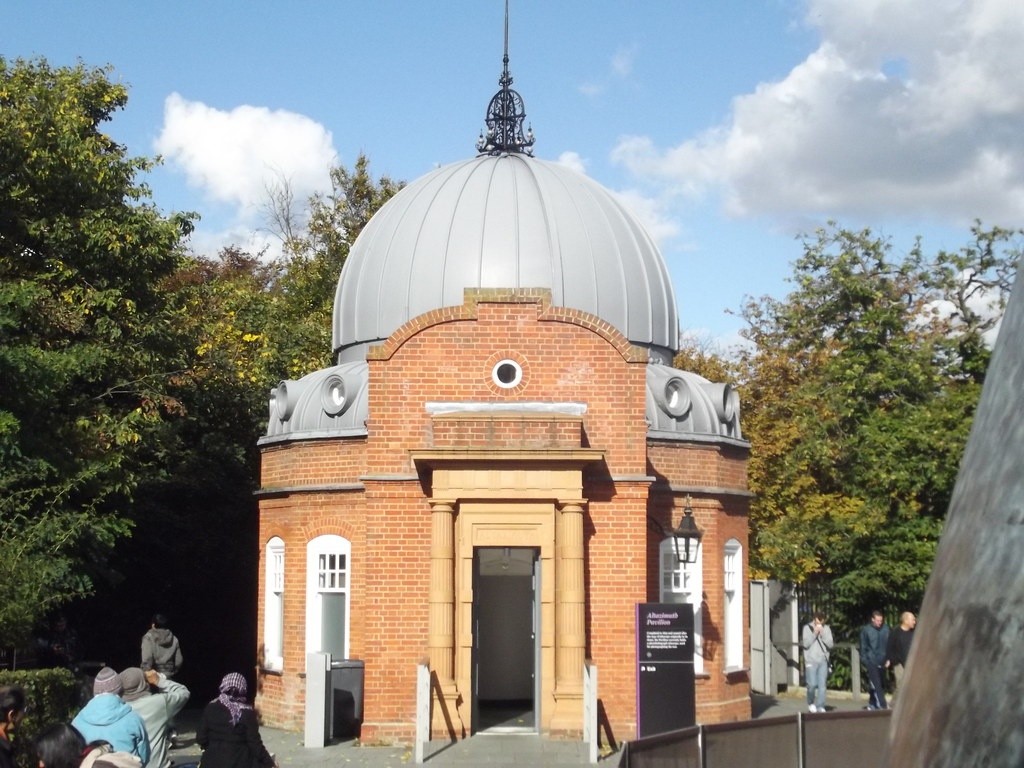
[828,662,834,673]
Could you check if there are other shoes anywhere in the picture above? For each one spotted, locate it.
[168,730,178,750]
[817,706,826,713]
[808,704,817,714]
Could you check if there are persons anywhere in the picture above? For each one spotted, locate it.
[139,615,183,767]
[884,610,916,693]
[856,611,892,709]
[801,613,833,712]
[196,671,279,768]
[0,663,191,768]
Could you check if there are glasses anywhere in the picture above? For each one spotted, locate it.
[5,705,28,713]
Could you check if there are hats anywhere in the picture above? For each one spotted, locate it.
[93,667,122,695]
[814,610,825,618]
[120,667,146,695]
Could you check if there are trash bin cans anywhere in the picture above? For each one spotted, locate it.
[331,659,364,739]
